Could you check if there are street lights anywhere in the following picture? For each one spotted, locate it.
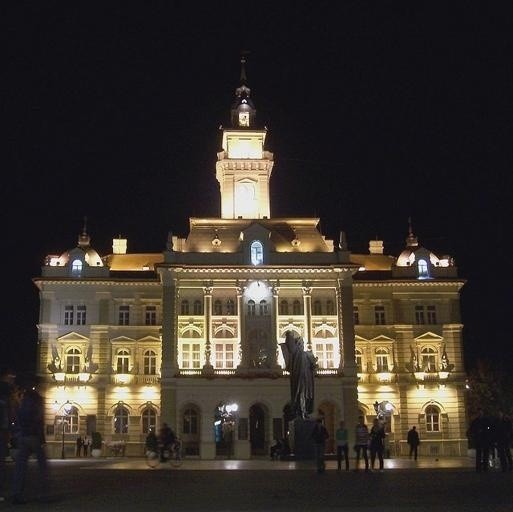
[217,401,238,459]
[373,401,392,419]
[52,400,72,459]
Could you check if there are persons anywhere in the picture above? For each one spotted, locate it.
[335,420,349,472]
[284,329,318,422]
[270,438,281,460]
[494,406,513,472]
[407,425,420,459]
[82,435,88,456]
[368,418,386,471]
[144,429,166,462]
[311,419,328,473]
[160,422,181,460]
[471,407,489,471]
[271,438,289,461]
[75,437,82,457]
[353,415,368,470]
[11,388,48,505]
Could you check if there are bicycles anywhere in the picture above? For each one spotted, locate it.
[145,437,184,468]
